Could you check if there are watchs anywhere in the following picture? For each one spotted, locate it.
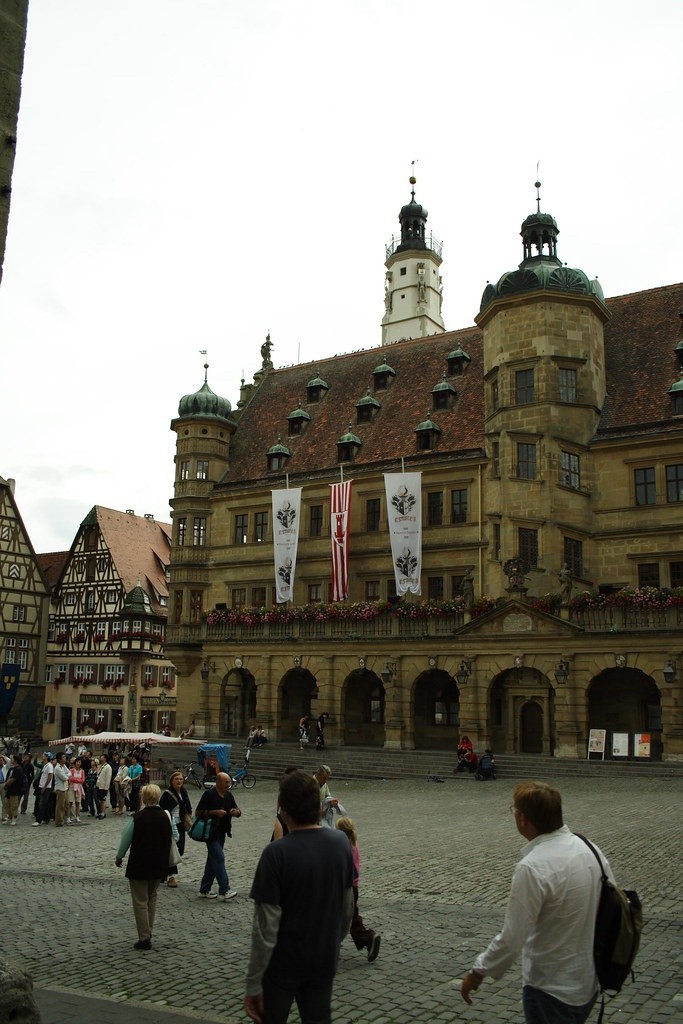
[237,810,240,815]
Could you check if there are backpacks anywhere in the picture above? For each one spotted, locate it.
[573,832,642,991]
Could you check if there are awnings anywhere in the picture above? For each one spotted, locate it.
[50,731,209,766]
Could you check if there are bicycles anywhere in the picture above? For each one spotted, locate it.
[164,760,202,790]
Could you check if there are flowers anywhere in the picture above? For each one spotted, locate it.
[53,676,65,690]
[201,584,683,628]
[101,679,113,690]
[70,676,93,689]
[111,679,124,692]
[72,633,84,643]
[92,631,163,647]
[143,679,155,691]
[78,718,108,735]
[55,632,68,645]
[161,680,174,689]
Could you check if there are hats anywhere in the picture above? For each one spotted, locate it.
[27,752,32,758]
[44,751,53,759]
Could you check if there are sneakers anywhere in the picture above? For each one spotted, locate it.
[220,890,237,899]
[198,890,217,898]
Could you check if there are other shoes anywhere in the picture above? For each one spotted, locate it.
[21,810,26,814]
[30,813,35,818]
[2,819,12,824]
[367,933,380,962]
[134,937,152,950]
[42,805,136,827]
[32,822,41,827]
[11,821,16,825]
[166,877,178,888]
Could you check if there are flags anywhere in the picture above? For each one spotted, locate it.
[272,469,424,603]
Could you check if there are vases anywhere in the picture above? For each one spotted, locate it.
[60,637,68,643]
[77,636,84,643]
[99,637,104,641]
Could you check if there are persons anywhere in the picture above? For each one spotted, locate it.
[0,711,498,959]
[243,770,360,1024]
[459,780,622,1024]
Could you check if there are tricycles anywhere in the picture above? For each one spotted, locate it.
[196,742,256,790]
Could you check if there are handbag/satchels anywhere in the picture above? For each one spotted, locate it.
[184,814,193,832]
[188,809,212,842]
[164,810,182,865]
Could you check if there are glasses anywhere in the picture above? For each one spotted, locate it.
[510,805,524,814]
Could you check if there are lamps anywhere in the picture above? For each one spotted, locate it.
[159,689,167,703]
[380,662,398,683]
[553,659,570,685]
[662,659,677,684]
[455,659,472,685]
